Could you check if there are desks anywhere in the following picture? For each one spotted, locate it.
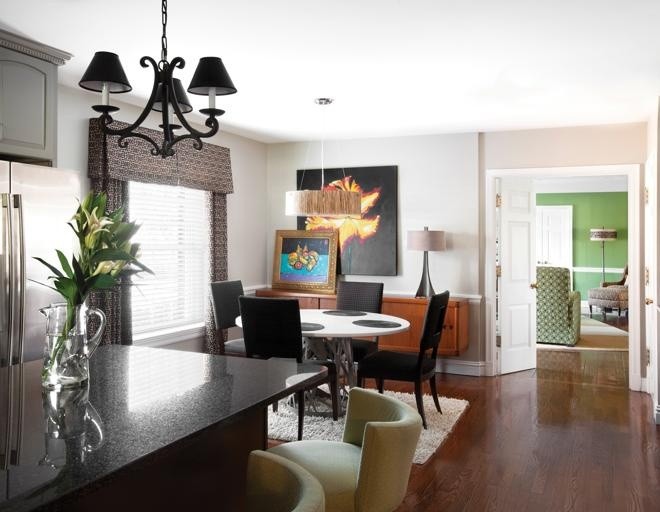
[231,301,412,417]
[1,340,328,512]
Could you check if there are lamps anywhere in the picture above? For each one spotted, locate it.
[588,225,617,283]
[75,2,240,156]
[407,226,448,298]
[285,90,365,218]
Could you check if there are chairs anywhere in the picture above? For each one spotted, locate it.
[236,296,339,443]
[267,383,424,511]
[242,446,327,510]
[211,280,278,359]
[601,267,627,317]
[304,277,392,397]
[356,288,449,428]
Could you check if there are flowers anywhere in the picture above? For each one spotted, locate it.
[28,190,154,386]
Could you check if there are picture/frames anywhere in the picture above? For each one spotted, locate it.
[272,228,339,295]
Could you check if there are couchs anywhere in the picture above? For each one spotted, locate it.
[588,285,628,328]
[537,267,581,347]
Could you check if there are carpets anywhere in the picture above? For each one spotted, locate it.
[536,312,629,351]
[264,380,470,465]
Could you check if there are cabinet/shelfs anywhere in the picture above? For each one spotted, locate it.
[255,288,470,355]
[1,46,61,165]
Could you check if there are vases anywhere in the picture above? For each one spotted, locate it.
[38,300,107,387]
[39,382,106,470]
